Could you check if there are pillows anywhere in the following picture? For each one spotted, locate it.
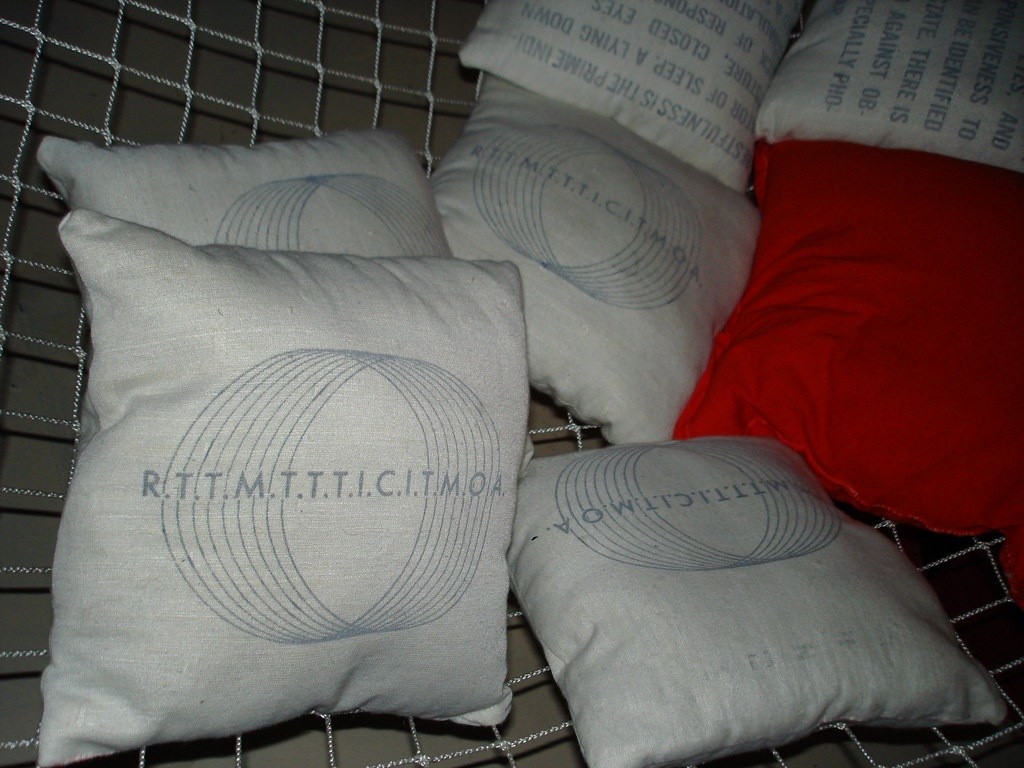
[754,0,1024,173]
[674,138,1024,608]
[431,72,761,444]
[459,0,805,194]
[35,209,531,768]
[36,128,455,258]
[506,437,1006,768]
